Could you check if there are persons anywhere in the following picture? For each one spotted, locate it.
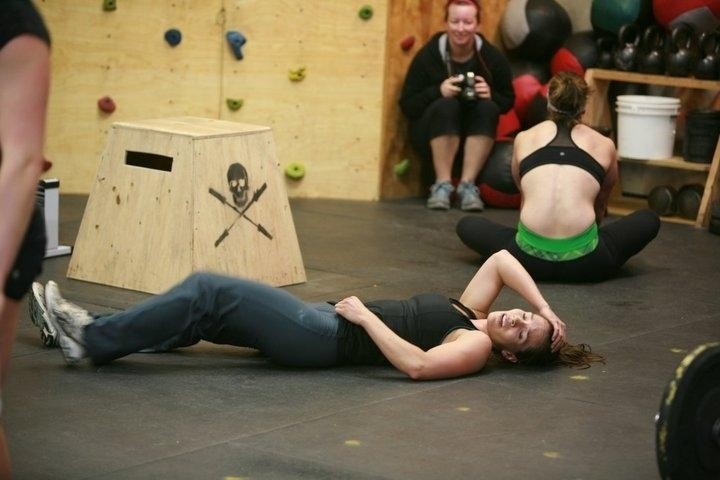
[399,1,516,211]
[455,74,661,284]
[0,1,53,480]
[26,250,606,382]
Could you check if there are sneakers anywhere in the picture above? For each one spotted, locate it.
[426,182,454,208]
[456,183,483,211]
[28,280,94,364]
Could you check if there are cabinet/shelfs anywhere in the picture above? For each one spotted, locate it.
[579,68,720,230]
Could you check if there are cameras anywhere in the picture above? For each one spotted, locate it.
[458,72,479,101]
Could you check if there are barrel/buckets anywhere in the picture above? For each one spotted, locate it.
[615,94,682,161]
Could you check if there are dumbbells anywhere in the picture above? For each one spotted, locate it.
[647,185,678,217]
[680,184,704,220]
